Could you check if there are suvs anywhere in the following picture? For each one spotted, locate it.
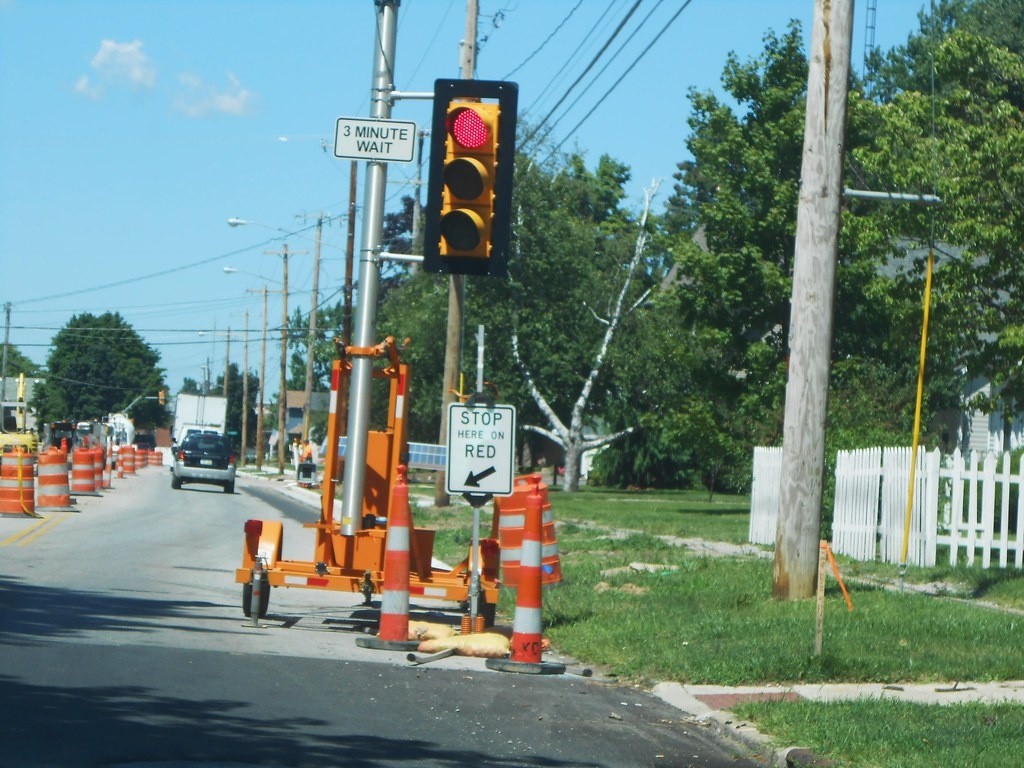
[169,432,237,492]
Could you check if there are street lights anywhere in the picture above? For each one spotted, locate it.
[196,132,359,485]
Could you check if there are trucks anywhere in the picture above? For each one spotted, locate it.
[172,391,227,457]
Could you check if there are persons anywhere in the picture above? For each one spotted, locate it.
[167,438,178,473]
[302,440,313,462]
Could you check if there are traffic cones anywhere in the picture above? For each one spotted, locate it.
[475,482,561,676]
[356,464,426,653]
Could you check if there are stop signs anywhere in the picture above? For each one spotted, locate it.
[445,402,515,498]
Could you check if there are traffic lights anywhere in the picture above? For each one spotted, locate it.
[438,101,501,257]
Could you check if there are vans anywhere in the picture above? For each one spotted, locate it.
[169,422,223,471]
